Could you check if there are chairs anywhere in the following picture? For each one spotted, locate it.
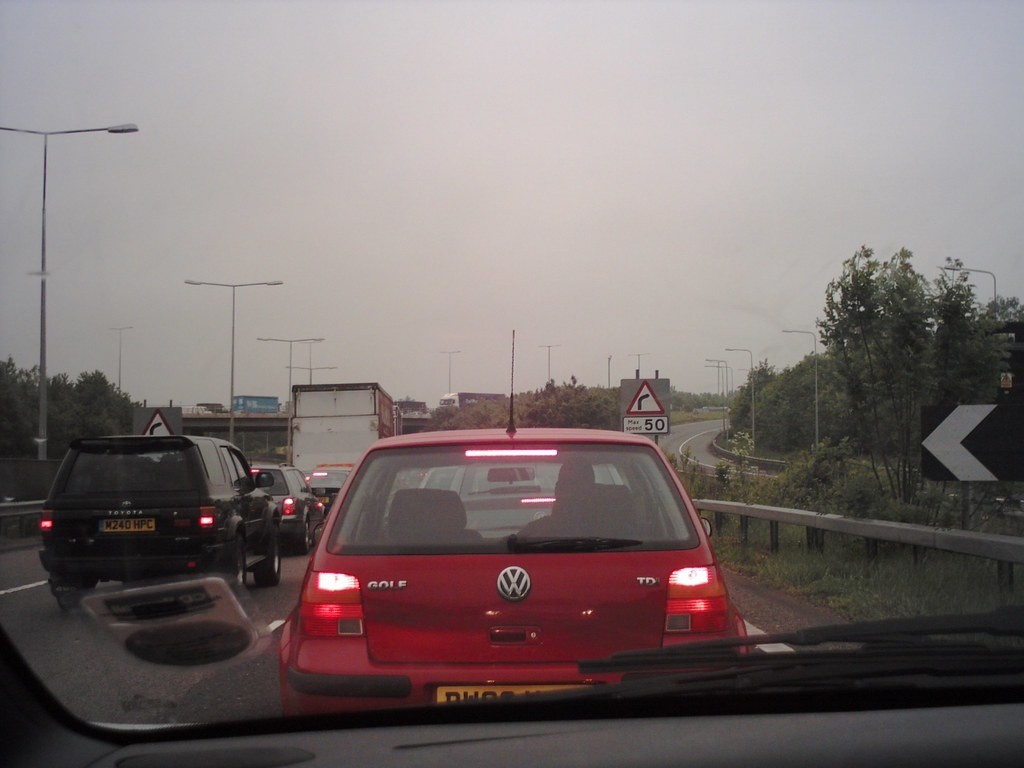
[550,485,649,540]
[378,489,484,541]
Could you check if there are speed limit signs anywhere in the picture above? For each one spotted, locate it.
[623,417,670,434]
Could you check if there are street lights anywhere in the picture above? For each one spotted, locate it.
[704,358,747,401]
[258,337,327,413]
[0,122,144,466]
[724,346,756,453]
[184,278,287,439]
[110,324,137,396]
[285,365,338,387]
[941,266,999,304]
[780,330,820,456]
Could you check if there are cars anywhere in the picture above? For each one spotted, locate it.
[277,425,749,726]
[303,469,351,519]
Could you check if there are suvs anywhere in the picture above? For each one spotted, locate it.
[37,432,286,608]
[247,462,326,556]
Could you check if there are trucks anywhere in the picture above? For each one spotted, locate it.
[290,381,405,505]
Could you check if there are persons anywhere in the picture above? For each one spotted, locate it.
[553,459,594,511]
